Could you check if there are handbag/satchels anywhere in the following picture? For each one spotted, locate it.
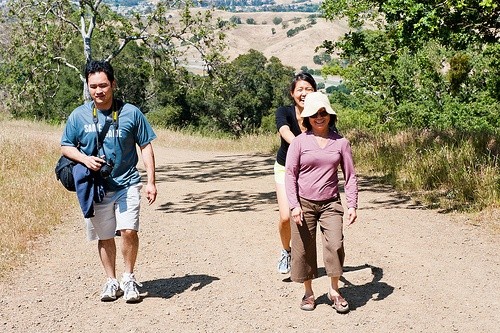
[55,155,76,192]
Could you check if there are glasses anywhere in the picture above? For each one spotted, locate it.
[310,110,328,118]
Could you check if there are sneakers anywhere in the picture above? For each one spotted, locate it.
[277,247,291,274]
[120,272,143,300]
[100,277,120,301]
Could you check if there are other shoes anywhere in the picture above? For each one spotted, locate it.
[327,288,349,312]
[300,294,316,310]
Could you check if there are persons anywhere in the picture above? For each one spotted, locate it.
[285,92,358,312]
[61,60,157,303]
[274,73,318,274]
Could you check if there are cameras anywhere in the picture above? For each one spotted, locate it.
[96,158,114,177]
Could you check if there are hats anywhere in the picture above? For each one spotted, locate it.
[300,92,336,118]
